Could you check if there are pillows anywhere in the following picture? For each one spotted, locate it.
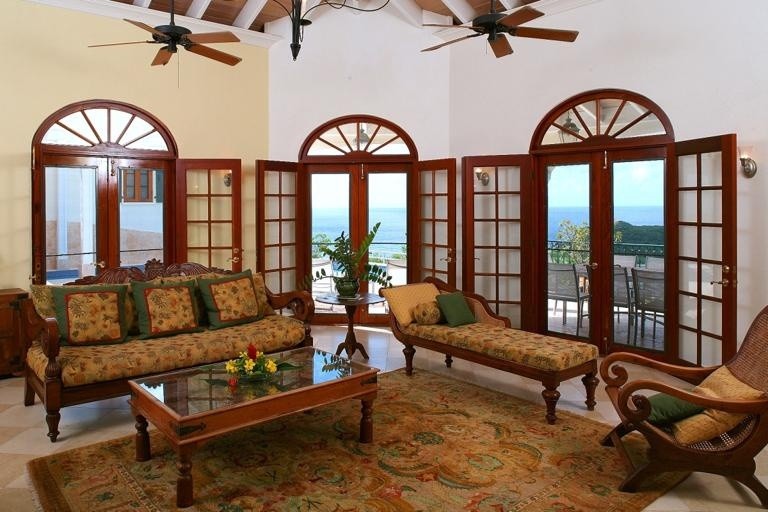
[411,298,444,326]
[26,277,162,343]
[380,279,445,326]
[162,270,276,318]
[434,289,476,329]
[48,281,132,348]
[197,267,265,330]
[126,277,205,339]
[639,388,709,428]
[670,366,765,448]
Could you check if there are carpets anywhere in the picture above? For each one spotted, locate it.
[23,364,698,510]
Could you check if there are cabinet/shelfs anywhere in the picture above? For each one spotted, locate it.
[0,286,32,382]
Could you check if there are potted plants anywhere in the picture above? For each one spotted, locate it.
[295,219,394,298]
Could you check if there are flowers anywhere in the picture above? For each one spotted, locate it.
[202,339,304,379]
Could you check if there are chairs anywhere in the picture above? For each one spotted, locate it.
[548,258,666,350]
[597,299,767,511]
[377,275,600,427]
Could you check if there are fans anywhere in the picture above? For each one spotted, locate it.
[414,0,580,64]
[88,0,245,73]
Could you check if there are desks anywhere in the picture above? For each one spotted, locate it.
[314,289,386,361]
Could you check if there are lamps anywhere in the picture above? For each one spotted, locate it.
[354,121,370,153]
[737,144,757,179]
[223,168,233,187]
[556,108,581,144]
[474,167,489,187]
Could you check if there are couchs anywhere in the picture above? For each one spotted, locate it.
[23,256,318,443]
